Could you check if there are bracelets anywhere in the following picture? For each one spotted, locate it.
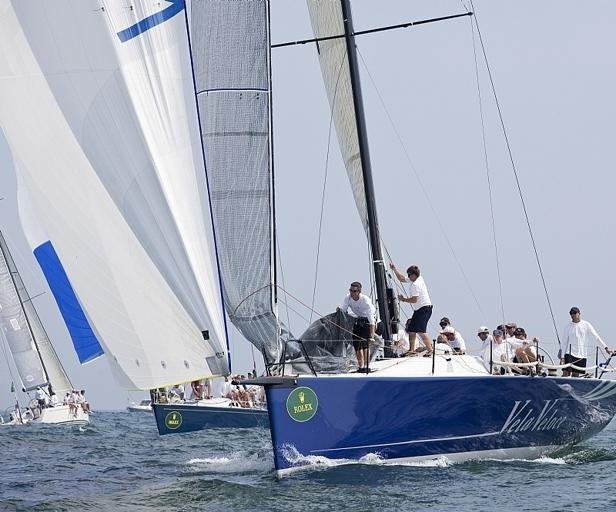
[402,298,406,302]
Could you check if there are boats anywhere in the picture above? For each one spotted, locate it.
[126,395,152,413]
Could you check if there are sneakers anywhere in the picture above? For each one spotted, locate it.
[422,349,433,357]
[403,351,416,357]
[357,366,371,373]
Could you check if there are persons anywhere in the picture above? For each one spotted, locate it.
[35,386,93,419]
[337,282,376,373]
[560,307,614,377]
[155,370,261,408]
[390,263,433,357]
[432,317,541,376]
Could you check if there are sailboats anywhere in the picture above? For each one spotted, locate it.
[1,0,297,439]
[232,2,616,487]
[1,10,95,432]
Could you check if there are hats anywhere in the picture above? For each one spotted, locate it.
[477,326,489,336]
[493,322,526,336]
[569,307,581,312]
[439,326,456,335]
[441,317,449,322]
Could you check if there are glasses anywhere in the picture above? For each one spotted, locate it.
[440,322,447,326]
[408,272,414,278]
[349,289,358,292]
[569,311,579,315]
